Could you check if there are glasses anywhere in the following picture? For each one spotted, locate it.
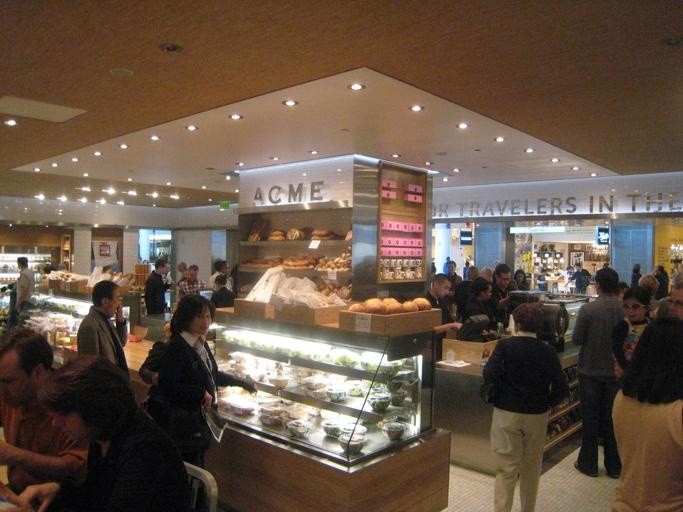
[623,303,648,310]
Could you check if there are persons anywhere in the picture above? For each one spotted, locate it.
[537,260,592,297]
[426,256,529,338]
[613,263,683,512]
[482,302,569,512]
[138,323,169,424]
[77,280,130,379]
[0,356,192,512]
[0,326,90,491]
[12,255,41,312]
[572,269,625,479]
[143,259,237,313]
[159,294,258,470]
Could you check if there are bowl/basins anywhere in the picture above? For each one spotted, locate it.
[217,330,408,456]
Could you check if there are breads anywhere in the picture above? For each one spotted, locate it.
[240,217,431,314]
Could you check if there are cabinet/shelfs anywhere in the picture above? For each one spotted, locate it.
[236,208,353,300]
[543,353,583,454]
[0,252,141,369]
[213,308,437,466]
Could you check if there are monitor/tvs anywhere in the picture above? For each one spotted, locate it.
[456,314,488,340]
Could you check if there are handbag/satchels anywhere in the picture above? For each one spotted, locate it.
[142,397,211,451]
[482,374,502,402]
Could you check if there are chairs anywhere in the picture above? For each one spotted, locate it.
[184,462,219,512]
